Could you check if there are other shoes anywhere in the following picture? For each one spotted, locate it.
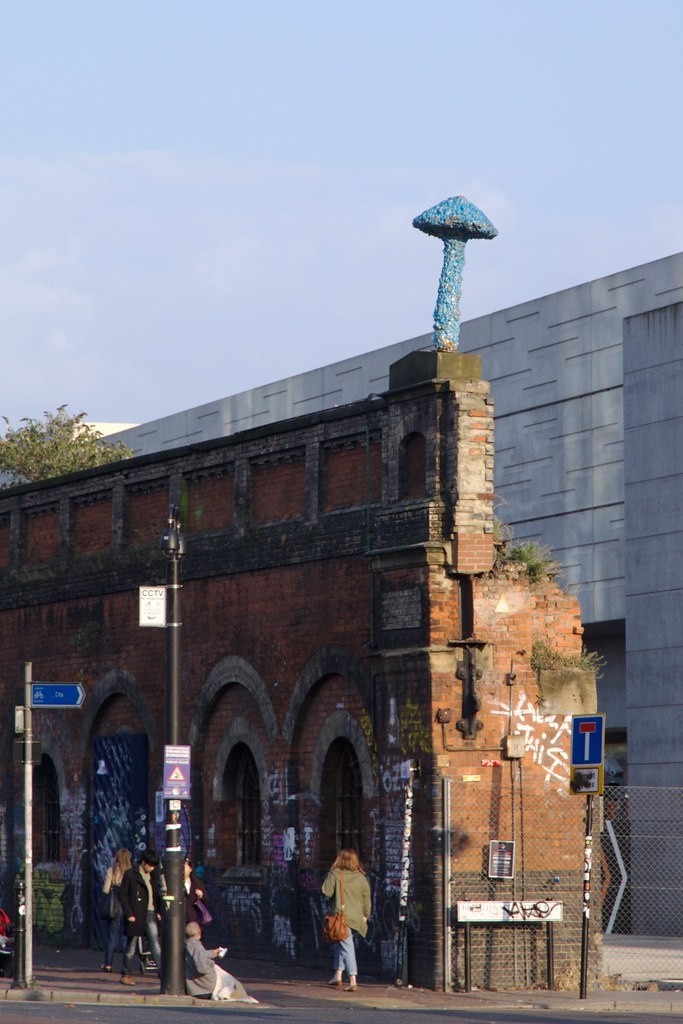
[119,977,136,986]
[328,979,342,986]
[101,964,112,973]
[341,985,359,992]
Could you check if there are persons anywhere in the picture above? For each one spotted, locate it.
[102,848,251,1001]
[321,848,372,992]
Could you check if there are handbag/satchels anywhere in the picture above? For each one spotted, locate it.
[95,868,121,921]
[192,896,213,930]
[322,910,349,944]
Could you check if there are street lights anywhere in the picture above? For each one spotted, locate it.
[159,499,191,998]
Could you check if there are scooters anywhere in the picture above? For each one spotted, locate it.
[0,905,17,978]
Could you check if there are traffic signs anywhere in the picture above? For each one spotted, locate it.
[30,681,86,708]
